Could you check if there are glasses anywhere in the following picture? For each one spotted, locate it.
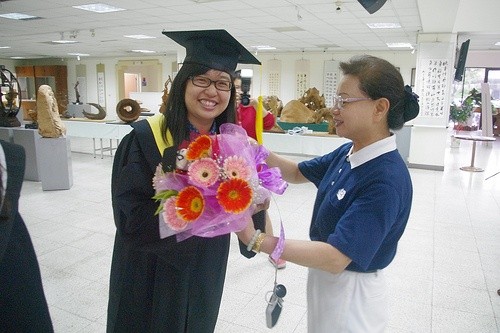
[332,94,375,107]
[187,75,234,91]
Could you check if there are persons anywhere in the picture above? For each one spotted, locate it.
[230,55,420,333]
[106,28,274,333]
[0,138,53,333]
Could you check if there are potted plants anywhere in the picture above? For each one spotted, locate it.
[450,88,495,148]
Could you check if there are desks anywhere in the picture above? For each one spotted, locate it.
[454,135,496,172]
[63,118,352,165]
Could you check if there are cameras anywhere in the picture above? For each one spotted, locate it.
[239,69,254,105]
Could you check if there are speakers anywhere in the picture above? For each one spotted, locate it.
[357,0,387,14]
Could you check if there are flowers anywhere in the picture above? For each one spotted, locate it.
[152,125,254,233]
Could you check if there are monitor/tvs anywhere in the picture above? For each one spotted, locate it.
[454,38,470,82]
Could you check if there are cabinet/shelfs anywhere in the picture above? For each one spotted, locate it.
[15,65,69,121]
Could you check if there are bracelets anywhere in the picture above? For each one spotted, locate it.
[252,233,265,255]
[246,229,261,251]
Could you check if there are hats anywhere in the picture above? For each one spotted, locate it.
[161,28,264,148]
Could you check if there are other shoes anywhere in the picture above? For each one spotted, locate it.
[267,254,287,270]
